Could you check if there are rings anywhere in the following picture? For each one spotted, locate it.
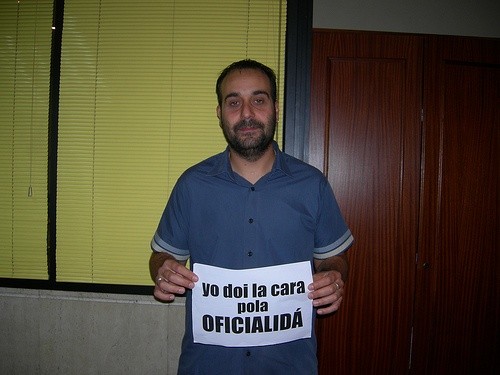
[156,278,163,286]
[333,282,340,290]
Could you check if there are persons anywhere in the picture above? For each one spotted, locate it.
[150,58,354,374]
